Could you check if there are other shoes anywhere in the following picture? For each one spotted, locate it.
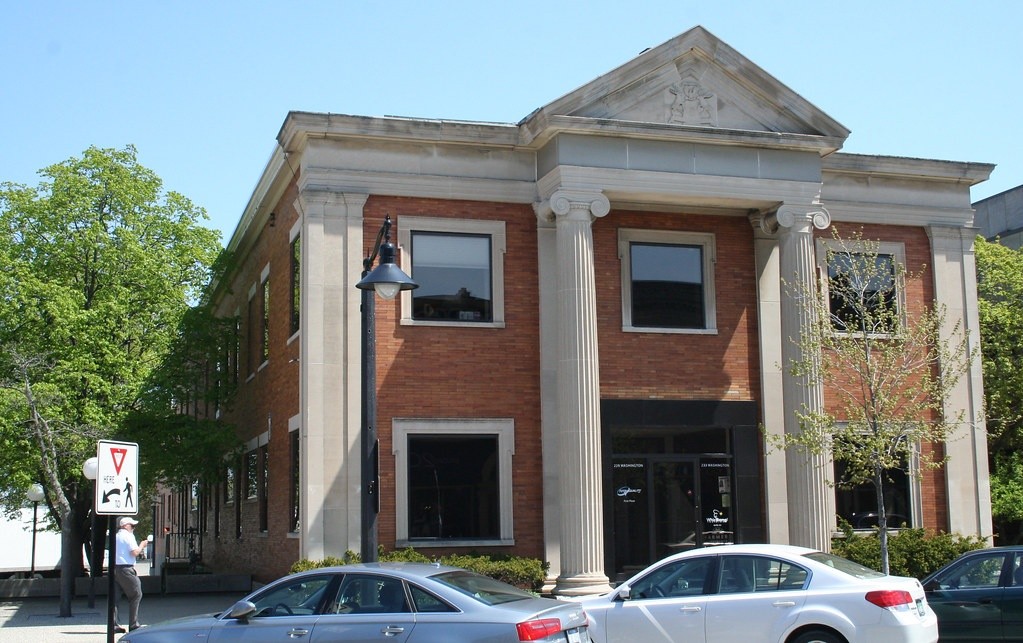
[129,624,147,634]
[113,626,125,633]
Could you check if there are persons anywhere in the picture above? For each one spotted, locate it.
[114,517,148,633]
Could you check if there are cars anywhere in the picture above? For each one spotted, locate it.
[579,544,938,643]
[920,546,1022,643]
[664,531,735,558]
[117,565,590,643]
[847,510,911,528]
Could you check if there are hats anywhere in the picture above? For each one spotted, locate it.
[119,517,140,527]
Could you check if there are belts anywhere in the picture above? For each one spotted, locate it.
[114,564,135,568]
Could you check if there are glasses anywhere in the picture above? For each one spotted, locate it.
[128,523,137,529]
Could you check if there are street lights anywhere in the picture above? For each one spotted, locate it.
[357,215,417,605]
[27,482,45,578]
[83,456,97,610]
